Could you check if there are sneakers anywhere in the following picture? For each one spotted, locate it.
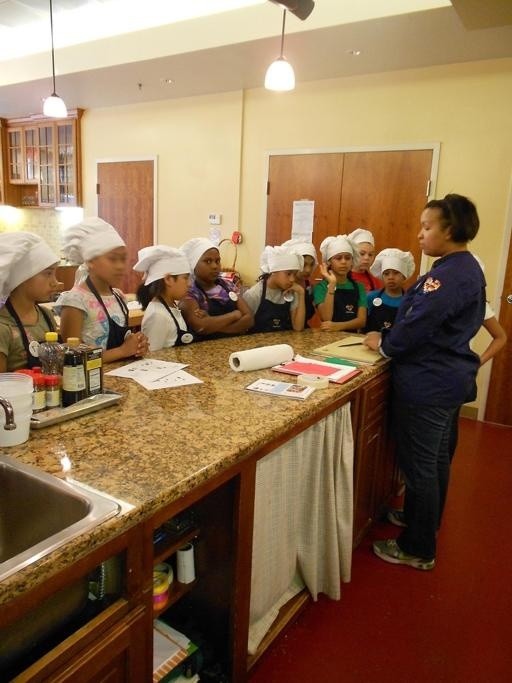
[373,540,435,570]
[387,512,406,527]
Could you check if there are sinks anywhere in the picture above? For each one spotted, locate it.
[0,453,137,587]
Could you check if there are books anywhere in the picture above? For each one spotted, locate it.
[311,335,383,366]
[153,619,198,682]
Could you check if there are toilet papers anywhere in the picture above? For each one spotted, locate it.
[229,344,295,372]
[176,543,196,585]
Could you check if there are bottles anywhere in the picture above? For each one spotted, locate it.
[62,337,84,407]
[32,367,47,413]
[45,363,62,409]
[39,332,65,374]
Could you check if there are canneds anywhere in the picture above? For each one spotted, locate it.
[16,369,61,414]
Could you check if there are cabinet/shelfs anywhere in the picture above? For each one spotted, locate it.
[0,108,85,210]
[0,370,405,682]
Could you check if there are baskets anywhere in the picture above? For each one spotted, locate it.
[218,239,242,288]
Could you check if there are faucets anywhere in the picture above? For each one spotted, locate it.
[0,396,17,430]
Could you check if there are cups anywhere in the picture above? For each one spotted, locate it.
[0,373,34,446]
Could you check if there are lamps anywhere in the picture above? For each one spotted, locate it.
[43,0,68,119]
[264,0,315,92]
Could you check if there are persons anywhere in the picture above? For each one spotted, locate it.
[363,192,487,570]
[0,232,63,373]
[178,236,255,339]
[448,251,507,465]
[54,216,152,364]
[348,228,384,293]
[313,235,367,333]
[132,245,196,352]
[281,238,322,328]
[243,245,306,333]
[360,248,416,335]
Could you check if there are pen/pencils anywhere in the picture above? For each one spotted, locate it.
[339,342,363,347]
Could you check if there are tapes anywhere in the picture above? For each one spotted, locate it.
[297,374,329,390]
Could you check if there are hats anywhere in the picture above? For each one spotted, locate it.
[133,238,219,285]
[64,217,125,263]
[261,228,415,281]
[0,232,60,296]
